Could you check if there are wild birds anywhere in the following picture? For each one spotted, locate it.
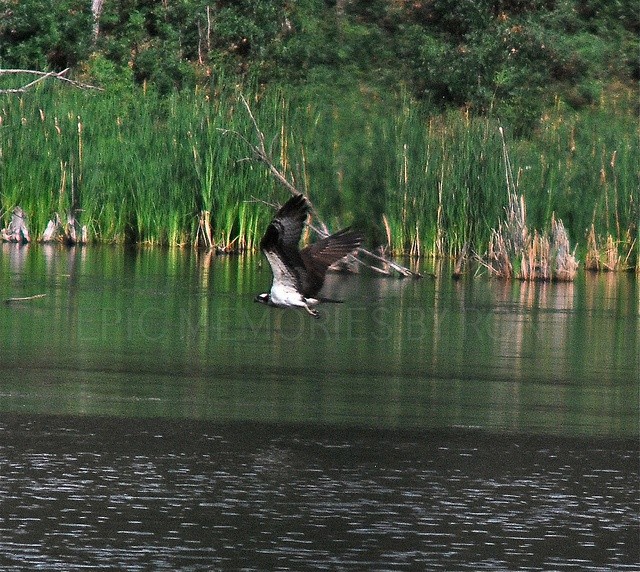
[253,193,366,321]
